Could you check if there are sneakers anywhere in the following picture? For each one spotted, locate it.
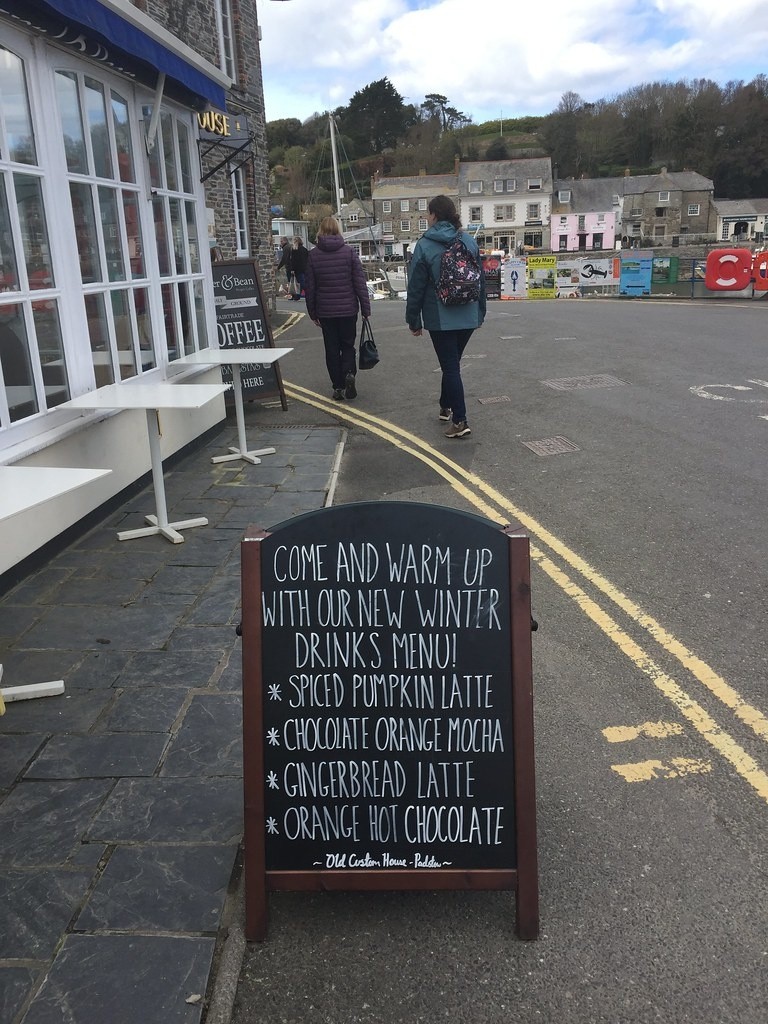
[444,419,471,438]
[332,388,345,400]
[344,370,358,399]
[438,408,452,420]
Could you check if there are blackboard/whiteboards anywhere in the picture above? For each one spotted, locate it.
[237,499,541,893]
[211,259,283,405]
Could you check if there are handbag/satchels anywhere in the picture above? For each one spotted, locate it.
[286,274,301,295]
[359,318,380,370]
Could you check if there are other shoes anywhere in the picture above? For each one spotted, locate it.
[287,296,298,300]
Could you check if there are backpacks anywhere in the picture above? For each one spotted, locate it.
[429,230,482,307]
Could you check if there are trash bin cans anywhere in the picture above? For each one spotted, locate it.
[672,236,679,247]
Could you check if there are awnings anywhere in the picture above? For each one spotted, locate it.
[46,0,232,147]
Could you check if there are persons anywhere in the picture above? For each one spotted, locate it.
[305,218,371,400]
[406,194,486,437]
[276,237,308,302]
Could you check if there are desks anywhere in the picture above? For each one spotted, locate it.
[58,384,232,544]
[5,385,68,409]
[0,466,114,718]
[41,349,177,383]
[167,348,295,465]
